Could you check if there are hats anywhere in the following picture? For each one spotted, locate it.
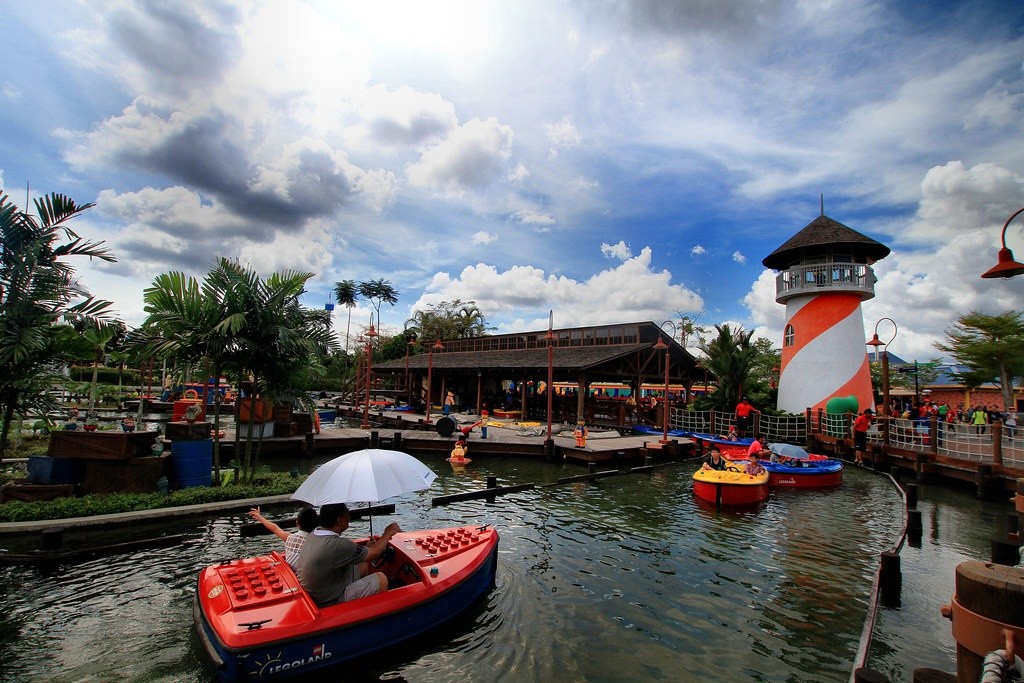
[742,396,750,400]
[864,409,875,414]
[448,392,454,396]
[1008,407,1015,410]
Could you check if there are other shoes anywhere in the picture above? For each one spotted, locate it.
[910,440,943,448]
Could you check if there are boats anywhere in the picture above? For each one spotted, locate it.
[391,406,622,440]
[694,434,755,452]
[188,524,499,682]
[733,453,844,487]
[691,459,770,509]
[123,380,237,413]
[359,399,394,407]
[635,426,690,438]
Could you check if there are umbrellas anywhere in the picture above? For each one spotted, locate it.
[291,448,437,540]
[768,443,808,459]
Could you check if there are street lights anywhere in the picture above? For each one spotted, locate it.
[404,332,416,387]
[426,329,444,423]
[865,318,897,442]
[651,320,677,443]
[355,310,379,428]
[542,310,560,446]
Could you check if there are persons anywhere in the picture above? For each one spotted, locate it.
[481,410,489,439]
[249,506,318,575]
[572,417,588,448]
[734,397,762,438]
[851,397,1018,467]
[481,387,698,422]
[702,426,797,476]
[297,503,401,608]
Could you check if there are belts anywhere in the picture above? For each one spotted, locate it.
[739,416,749,419]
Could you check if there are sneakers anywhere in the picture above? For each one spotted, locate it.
[854,459,864,468]
